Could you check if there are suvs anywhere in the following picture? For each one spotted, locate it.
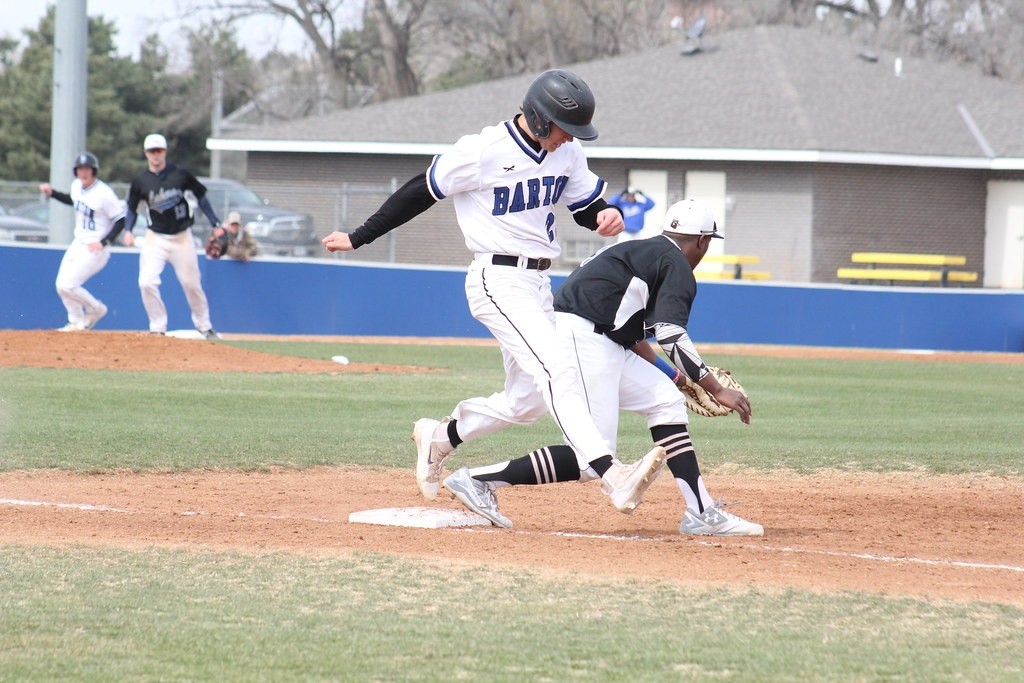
[191,176,322,258]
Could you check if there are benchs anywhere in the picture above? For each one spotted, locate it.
[838,252,978,288]
[692,255,772,280]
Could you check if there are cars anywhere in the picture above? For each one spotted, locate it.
[1,197,47,241]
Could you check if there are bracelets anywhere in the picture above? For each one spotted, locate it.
[671,368,681,383]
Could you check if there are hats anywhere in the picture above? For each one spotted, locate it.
[228,211,240,224]
[664,199,725,241]
[144,134,167,151]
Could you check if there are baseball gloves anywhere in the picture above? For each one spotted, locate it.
[206,227,228,258]
[679,364,749,418]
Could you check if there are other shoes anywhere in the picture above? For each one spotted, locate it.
[85,301,107,329]
[200,329,218,342]
[56,322,80,332]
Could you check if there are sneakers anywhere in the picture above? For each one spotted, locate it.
[601,447,667,514]
[412,417,449,502]
[441,466,512,528]
[680,500,764,536]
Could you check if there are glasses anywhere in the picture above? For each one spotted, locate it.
[148,148,166,156]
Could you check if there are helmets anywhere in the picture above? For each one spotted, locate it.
[523,70,598,142]
[73,153,99,175]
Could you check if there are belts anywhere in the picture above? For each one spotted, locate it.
[492,252,552,271]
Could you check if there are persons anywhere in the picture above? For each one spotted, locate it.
[409,200,764,536]
[40,152,125,331]
[609,187,655,243]
[320,70,667,515]
[124,134,221,340]
[206,211,258,262]
